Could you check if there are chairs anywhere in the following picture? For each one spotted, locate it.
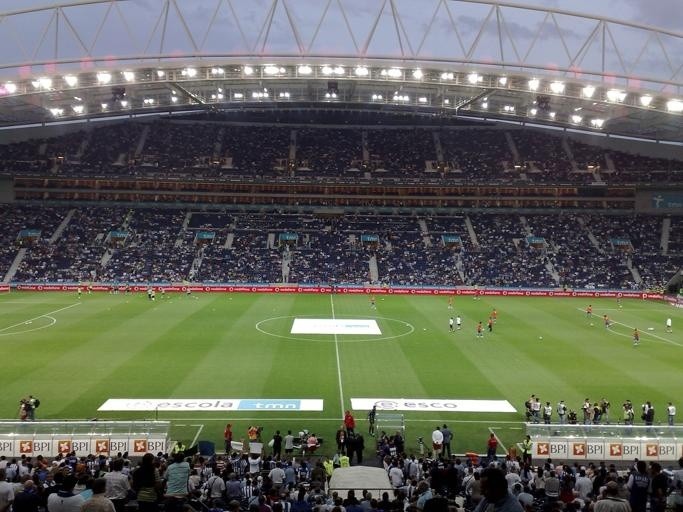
[2,203,683,289]
[2,107,683,187]
